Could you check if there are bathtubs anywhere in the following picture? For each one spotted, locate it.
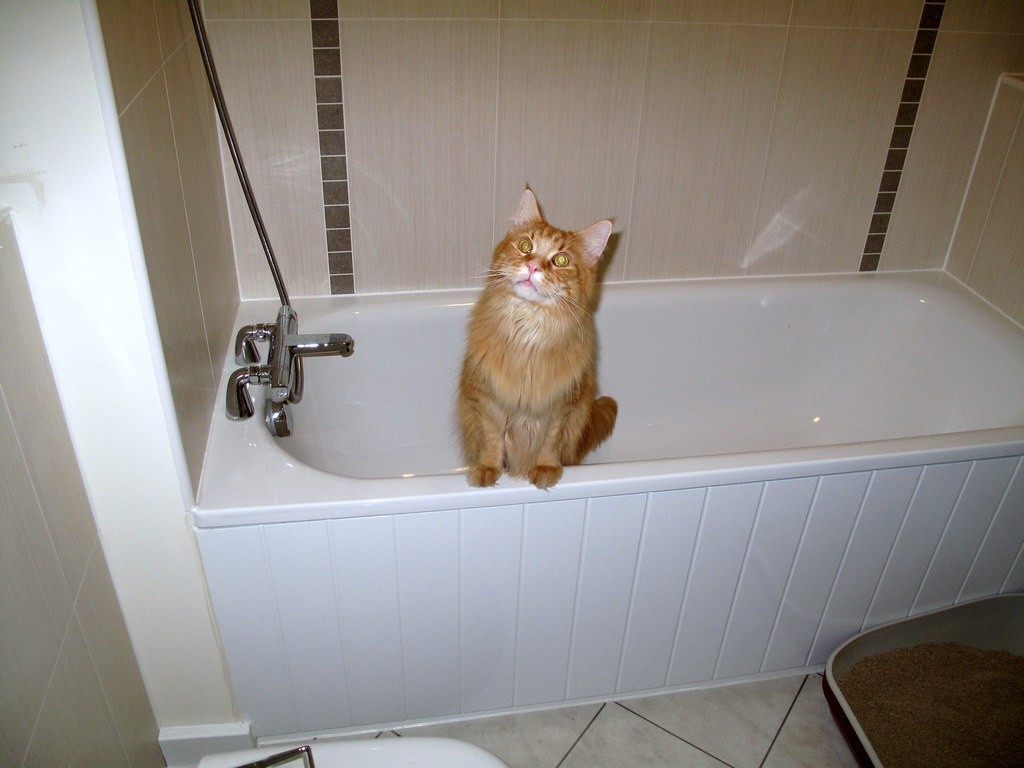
[196,269,1024,748]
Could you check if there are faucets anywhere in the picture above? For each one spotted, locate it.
[297,333,354,358]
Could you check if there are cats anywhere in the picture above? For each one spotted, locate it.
[446,189,619,489]
